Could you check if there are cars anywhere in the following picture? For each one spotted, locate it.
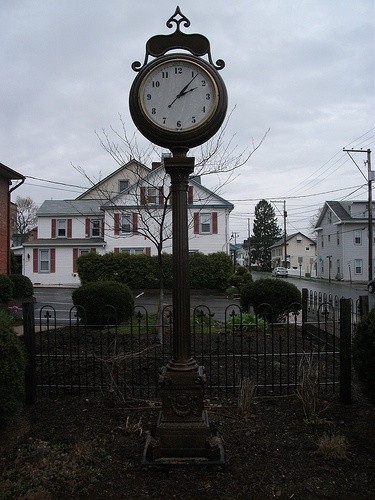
[272,267,288,278]
